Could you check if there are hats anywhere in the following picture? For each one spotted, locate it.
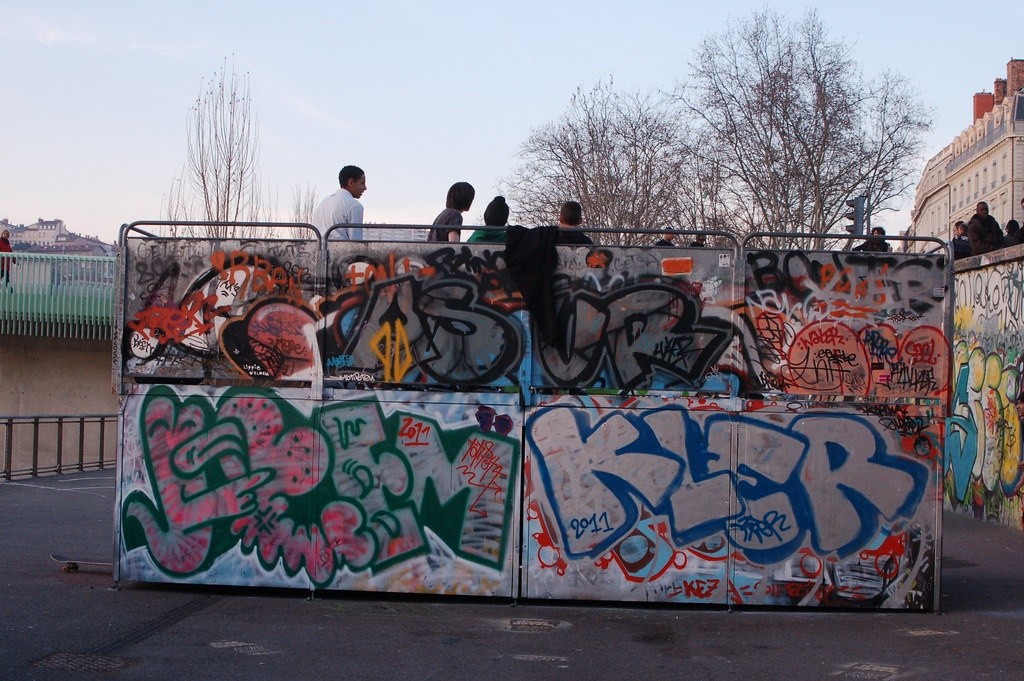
[484,196,509,226]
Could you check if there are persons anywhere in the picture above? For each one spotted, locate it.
[311,166,367,240]
[1003,220,1020,248]
[952,221,972,260]
[427,182,475,242]
[559,201,594,244]
[967,201,1003,256]
[0,230,19,294]
[689,234,705,247]
[655,227,675,246]
[467,196,509,242]
[852,227,887,252]
[1016,198,1024,244]
